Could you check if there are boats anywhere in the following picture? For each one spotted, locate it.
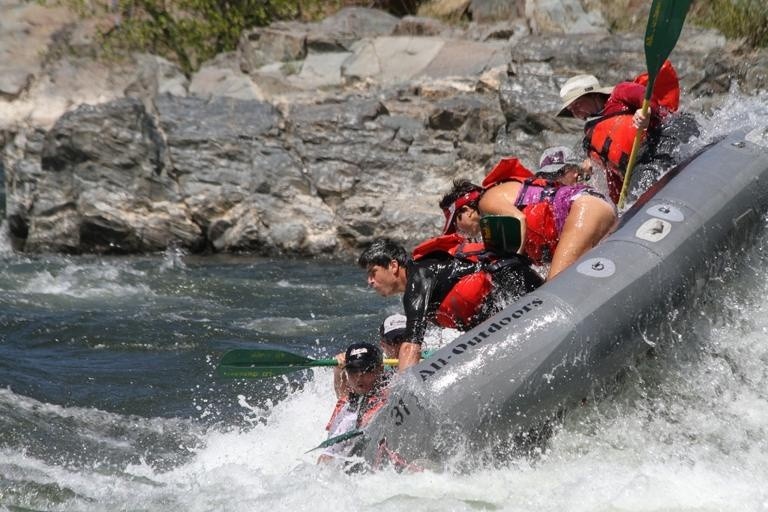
[347,125,768,476]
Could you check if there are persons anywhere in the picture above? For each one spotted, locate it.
[555,75,700,203]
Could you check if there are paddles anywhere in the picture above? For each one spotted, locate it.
[219,349,437,379]
[617,0,693,210]
[481,216,520,256]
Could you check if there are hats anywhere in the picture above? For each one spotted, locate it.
[554,73,615,118]
[378,311,410,341]
[341,341,384,371]
[440,190,482,236]
[534,144,582,176]
